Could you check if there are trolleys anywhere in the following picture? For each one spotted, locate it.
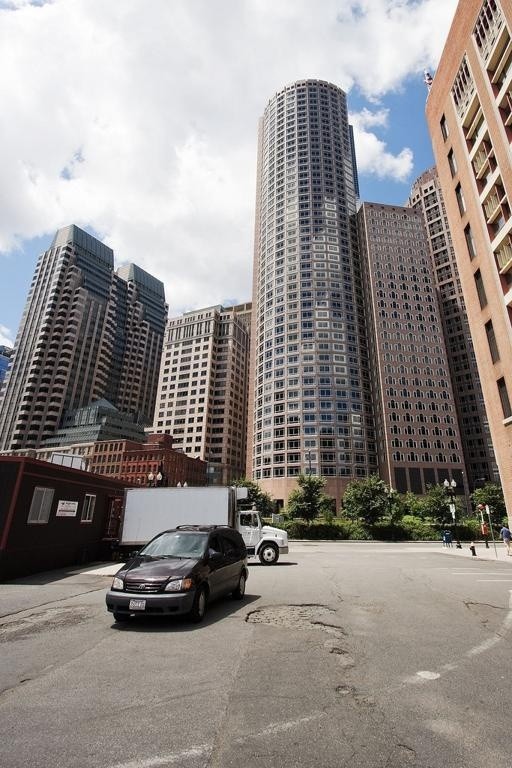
[442,530,454,548]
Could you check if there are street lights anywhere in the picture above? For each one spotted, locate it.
[176,481,189,487]
[473,477,487,491]
[147,471,164,487]
[307,448,313,476]
[443,477,462,548]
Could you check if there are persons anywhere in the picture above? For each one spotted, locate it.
[498,524,511,556]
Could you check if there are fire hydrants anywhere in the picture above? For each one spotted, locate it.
[470,542,477,557]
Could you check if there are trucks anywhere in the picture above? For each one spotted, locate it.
[116,484,291,566]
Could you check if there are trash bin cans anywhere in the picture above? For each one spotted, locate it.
[443,530,452,547]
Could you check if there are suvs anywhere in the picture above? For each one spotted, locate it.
[102,521,252,626]
